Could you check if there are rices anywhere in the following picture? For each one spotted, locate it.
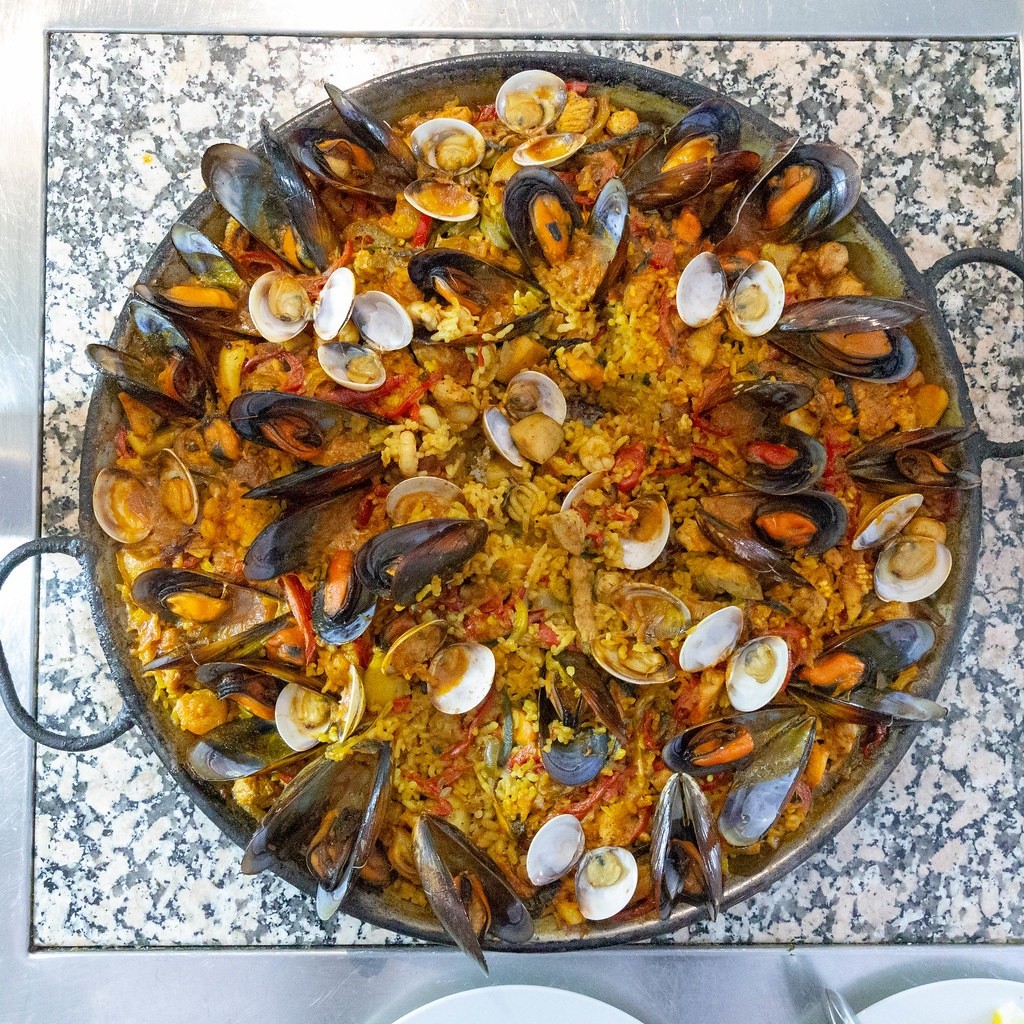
[115,96,915,915]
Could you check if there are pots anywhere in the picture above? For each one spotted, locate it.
[0,50,1024,954]
